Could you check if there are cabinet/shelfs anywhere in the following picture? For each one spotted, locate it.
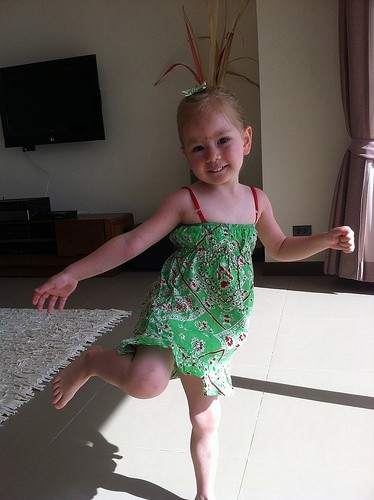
[0,213,133,277]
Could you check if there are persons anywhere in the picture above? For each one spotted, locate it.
[32,87,356,500]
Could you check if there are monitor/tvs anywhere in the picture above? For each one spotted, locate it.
[0,54,106,148]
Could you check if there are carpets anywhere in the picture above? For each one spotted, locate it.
[0,307,133,425]
